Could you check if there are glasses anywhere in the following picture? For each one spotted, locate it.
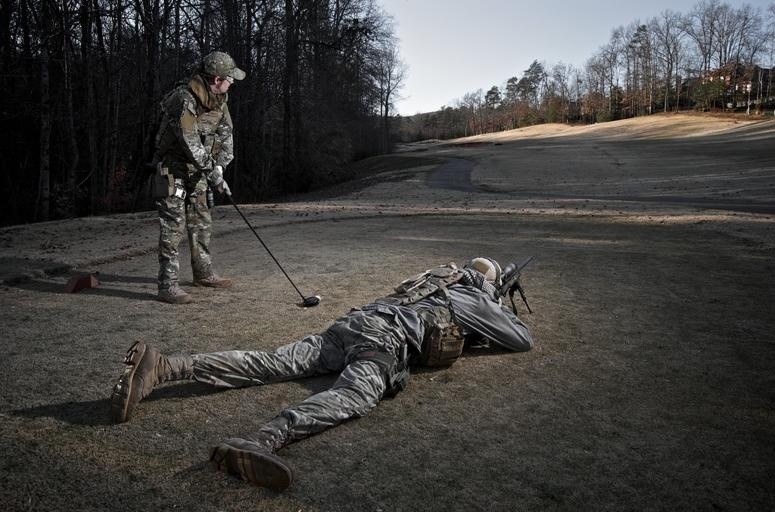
[220,77,234,84]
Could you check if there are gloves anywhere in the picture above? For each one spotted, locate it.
[215,166,232,196]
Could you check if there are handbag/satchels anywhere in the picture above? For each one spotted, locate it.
[420,307,465,366]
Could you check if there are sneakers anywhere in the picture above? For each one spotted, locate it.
[193,274,232,289]
[157,286,192,304]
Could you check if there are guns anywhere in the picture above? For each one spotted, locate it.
[498,256,536,316]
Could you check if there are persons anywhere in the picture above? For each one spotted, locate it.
[108,254,535,494]
[148,50,248,306]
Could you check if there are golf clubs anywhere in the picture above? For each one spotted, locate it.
[224,185,322,305]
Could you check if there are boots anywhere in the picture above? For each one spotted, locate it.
[212,414,295,489]
[110,341,194,422]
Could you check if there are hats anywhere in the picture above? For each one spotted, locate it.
[471,256,503,286]
[202,51,246,81]
